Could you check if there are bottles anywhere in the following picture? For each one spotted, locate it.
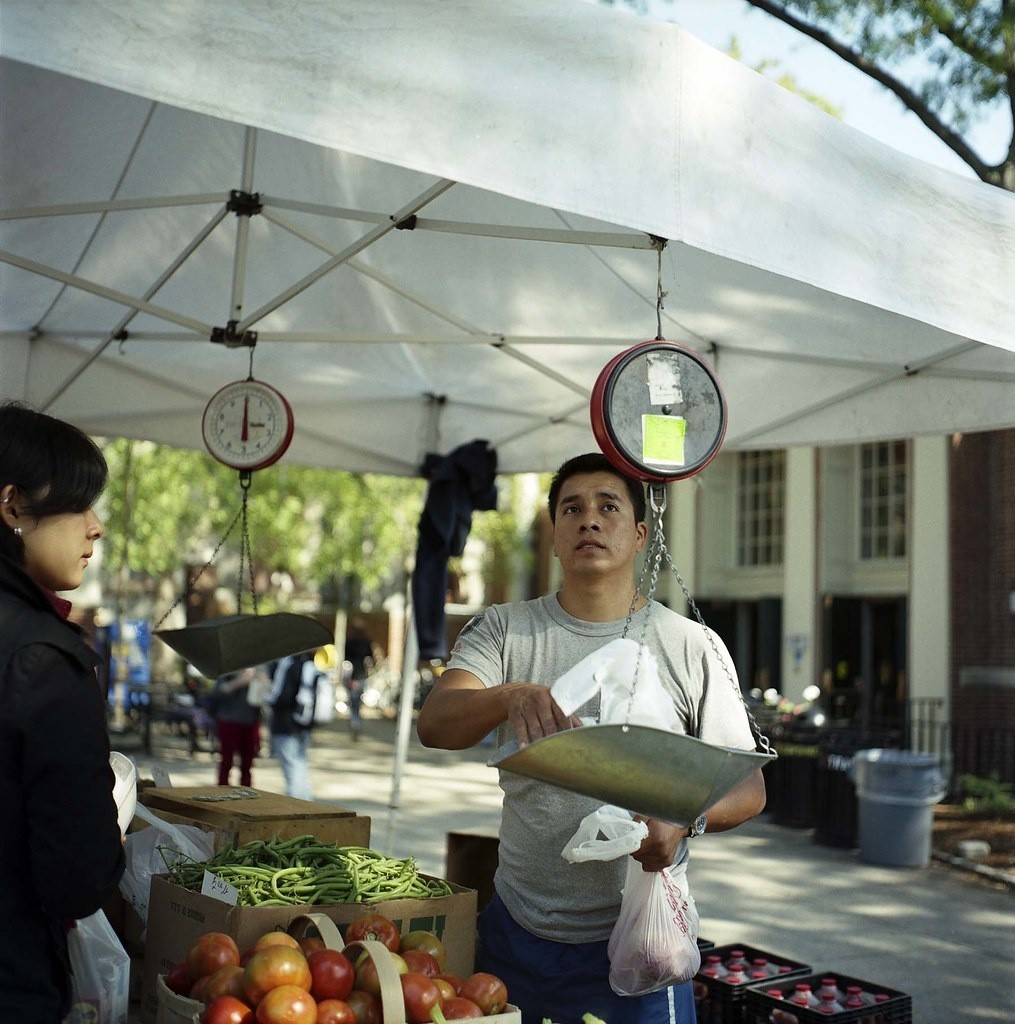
[694,950,891,1024]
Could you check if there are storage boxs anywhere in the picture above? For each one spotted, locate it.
[693,938,912,1024]
[154,973,521,1024]
[128,785,371,856]
[143,873,479,980]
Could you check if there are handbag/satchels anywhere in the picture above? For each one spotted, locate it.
[63,909,131,1024]
[560,805,701,995]
[116,801,215,946]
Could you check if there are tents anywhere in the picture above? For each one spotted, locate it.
[0,2,1015,481]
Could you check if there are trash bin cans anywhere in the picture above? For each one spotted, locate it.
[852,746,948,869]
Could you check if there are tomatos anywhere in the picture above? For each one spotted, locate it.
[163,912,506,1024]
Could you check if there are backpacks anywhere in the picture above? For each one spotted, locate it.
[290,654,336,729]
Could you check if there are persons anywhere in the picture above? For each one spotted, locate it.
[416,450,770,1024]
[166,683,182,736]
[183,677,207,694]
[344,669,368,743]
[0,400,126,1023]
[265,656,319,802]
[207,671,261,787]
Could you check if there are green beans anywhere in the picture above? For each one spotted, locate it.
[161,830,455,907]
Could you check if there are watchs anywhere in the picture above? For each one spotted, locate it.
[693,814,709,840]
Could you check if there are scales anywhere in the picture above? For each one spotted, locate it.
[151,375,335,681]
[485,339,781,830]
